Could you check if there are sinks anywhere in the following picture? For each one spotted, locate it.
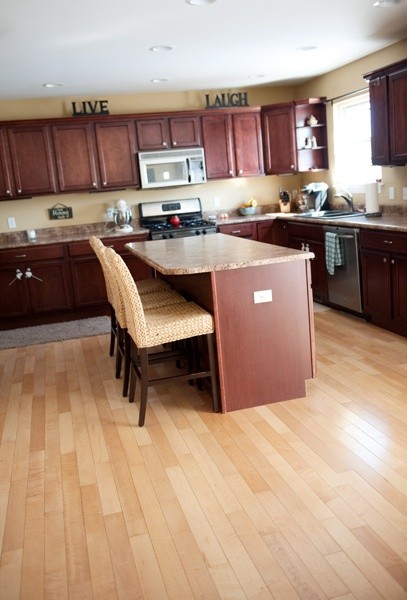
[302,210,360,219]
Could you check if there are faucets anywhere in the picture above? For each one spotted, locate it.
[333,191,353,212]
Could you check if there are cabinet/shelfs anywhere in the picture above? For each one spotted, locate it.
[362,227,406,338]
[0,241,73,321]
[261,98,330,175]
[288,223,328,301]
[368,66,407,165]
[52,116,139,194]
[135,110,202,153]
[218,220,288,248]
[202,107,265,180]
[68,232,154,311]
[0,118,58,202]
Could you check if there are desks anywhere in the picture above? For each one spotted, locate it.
[125,233,315,415]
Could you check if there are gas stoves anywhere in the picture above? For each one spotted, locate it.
[140,218,217,239]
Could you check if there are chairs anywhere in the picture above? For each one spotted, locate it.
[96,246,187,397]
[89,235,171,370]
[104,248,220,427]
